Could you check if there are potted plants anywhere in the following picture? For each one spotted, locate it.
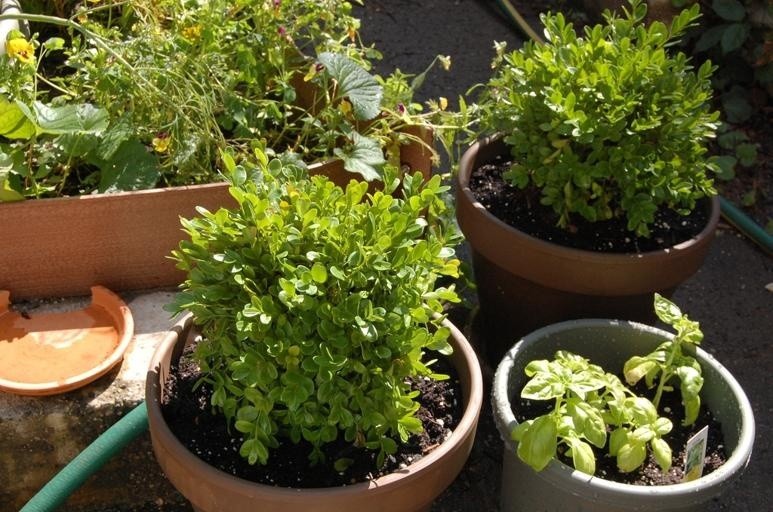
[486,288,756,511]
[144,133,485,512]
[454,0,721,352]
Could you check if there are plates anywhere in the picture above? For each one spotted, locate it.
[0,285,136,397]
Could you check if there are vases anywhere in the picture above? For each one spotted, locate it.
[0,73,394,299]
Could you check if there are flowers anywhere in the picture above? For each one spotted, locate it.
[0,0,466,198]
